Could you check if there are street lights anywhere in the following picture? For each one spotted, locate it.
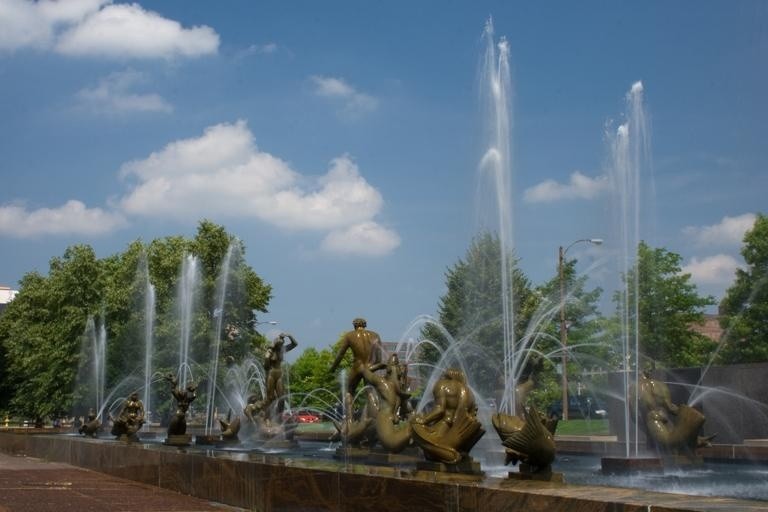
[555,235,609,419]
[240,319,277,359]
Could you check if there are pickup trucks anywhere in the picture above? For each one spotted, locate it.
[546,395,611,421]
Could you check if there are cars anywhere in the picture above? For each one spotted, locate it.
[278,395,499,423]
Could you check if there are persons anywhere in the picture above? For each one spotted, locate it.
[168,375,198,436]
[34,412,43,429]
[120,390,146,433]
[361,358,418,450]
[71,414,82,428]
[243,395,270,432]
[330,318,381,428]
[491,369,560,463]
[410,367,479,468]
[260,331,296,424]
[626,366,719,447]
[81,407,97,438]
